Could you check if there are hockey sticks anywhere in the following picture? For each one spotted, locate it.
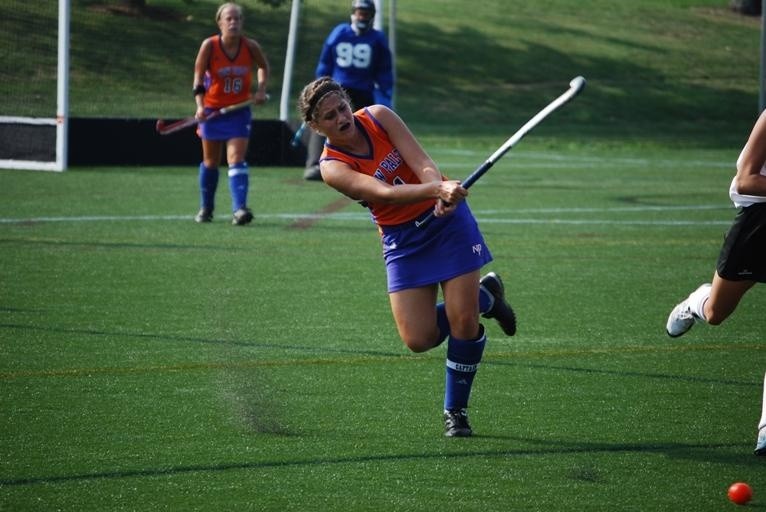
[156,94,271,136]
[415,76,585,231]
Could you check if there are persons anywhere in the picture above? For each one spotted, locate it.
[188,3,272,226]
[295,76,517,437]
[663,107,766,466]
[304,1,394,182]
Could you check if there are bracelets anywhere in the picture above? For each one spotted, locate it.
[191,83,205,100]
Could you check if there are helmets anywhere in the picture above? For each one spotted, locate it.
[351,0,376,31]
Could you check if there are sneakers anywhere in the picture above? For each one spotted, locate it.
[194,207,214,223]
[480,271,517,336]
[443,407,472,437]
[665,281,711,337]
[232,208,251,225]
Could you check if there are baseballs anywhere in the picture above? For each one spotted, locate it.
[728,482,752,504]
